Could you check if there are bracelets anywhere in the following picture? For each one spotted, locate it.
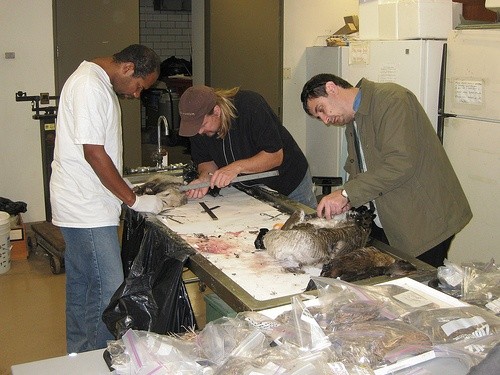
[340,186,350,203]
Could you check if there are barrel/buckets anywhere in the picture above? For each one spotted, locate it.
[0,212,14,274]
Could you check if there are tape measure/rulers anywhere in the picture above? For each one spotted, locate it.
[179,170,279,194]
[200,201,219,219]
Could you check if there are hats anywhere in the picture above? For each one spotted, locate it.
[178,85,217,136]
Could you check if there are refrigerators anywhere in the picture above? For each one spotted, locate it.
[306,39,445,229]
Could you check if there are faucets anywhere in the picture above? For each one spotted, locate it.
[157,115,169,171]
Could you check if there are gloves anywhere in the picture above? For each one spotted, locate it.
[127,195,163,214]
[122,178,135,188]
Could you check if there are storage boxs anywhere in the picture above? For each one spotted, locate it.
[324,0,464,42]
[9,214,29,261]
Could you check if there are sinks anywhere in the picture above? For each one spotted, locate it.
[125,169,185,186]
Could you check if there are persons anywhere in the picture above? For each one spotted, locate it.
[299,72,461,270]
[49,45,165,355]
[177,84,319,210]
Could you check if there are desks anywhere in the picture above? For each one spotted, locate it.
[117,165,437,327]
[10,267,500,375]
